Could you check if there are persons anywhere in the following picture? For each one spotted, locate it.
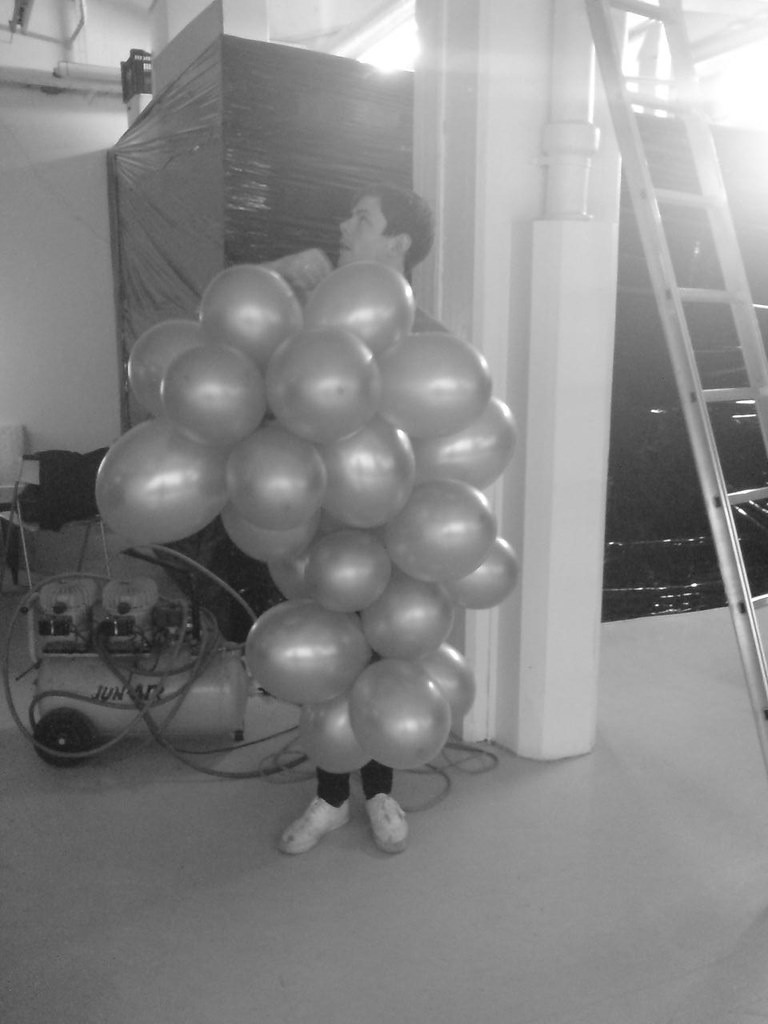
[274,187,451,852]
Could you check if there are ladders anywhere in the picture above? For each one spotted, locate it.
[587,1,768,773]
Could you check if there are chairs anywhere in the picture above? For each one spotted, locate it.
[1,427,40,577]
[3,451,111,586]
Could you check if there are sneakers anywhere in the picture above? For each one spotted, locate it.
[276,796,351,854]
[366,793,408,853]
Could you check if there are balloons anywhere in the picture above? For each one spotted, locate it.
[95,261,520,774]
